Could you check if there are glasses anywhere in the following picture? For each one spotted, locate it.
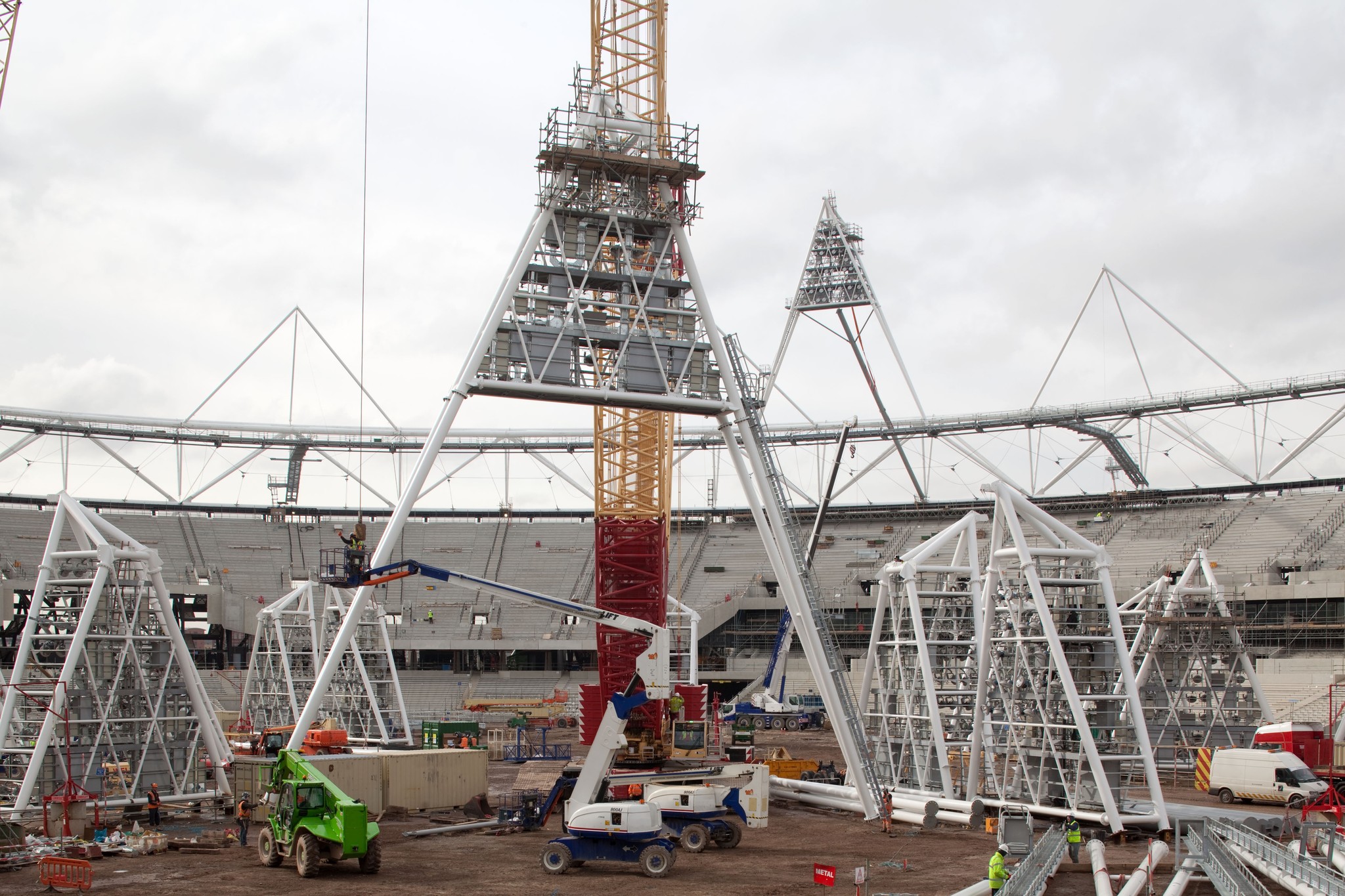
[245,795,250,798]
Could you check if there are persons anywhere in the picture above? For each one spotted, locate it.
[1059,812,1080,863]
[881,785,892,833]
[458,734,468,748]
[470,737,477,746]
[338,531,364,564]
[988,844,1012,896]
[668,692,684,730]
[290,790,306,804]
[147,783,162,831]
[628,784,642,801]
[1107,512,1111,519]
[428,610,433,623]
[238,792,261,847]
[725,594,730,602]
[679,725,696,740]
[1096,512,1102,517]
[30,741,36,746]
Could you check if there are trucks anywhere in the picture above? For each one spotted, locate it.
[1248,720,1345,807]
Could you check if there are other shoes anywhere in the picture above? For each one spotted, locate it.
[880,830,886,832]
[243,844,252,847]
[157,827,163,831]
[887,830,891,833]
[149,827,155,831]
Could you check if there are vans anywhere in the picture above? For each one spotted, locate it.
[1207,745,1332,809]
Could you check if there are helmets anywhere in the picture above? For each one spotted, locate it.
[882,785,889,789]
[429,610,432,612]
[1107,512,1110,514]
[894,555,899,559]
[998,844,1009,853]
[1067,811,1075,817]
[349,532,357,539]
[152,783,158,787]
[241,791,250,797]
[674,692,680,697]
[686,725,692,728]
[1101,512,1103,514]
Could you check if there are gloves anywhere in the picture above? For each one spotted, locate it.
[1006,877,1012,884]
[157,802,161,806]
[630,794,633,798]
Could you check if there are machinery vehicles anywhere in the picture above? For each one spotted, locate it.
[718,414,860,732]
[462,688,578,729]
[232,718,354,759]
[315,547,773,880]
[258,749,382,878]
